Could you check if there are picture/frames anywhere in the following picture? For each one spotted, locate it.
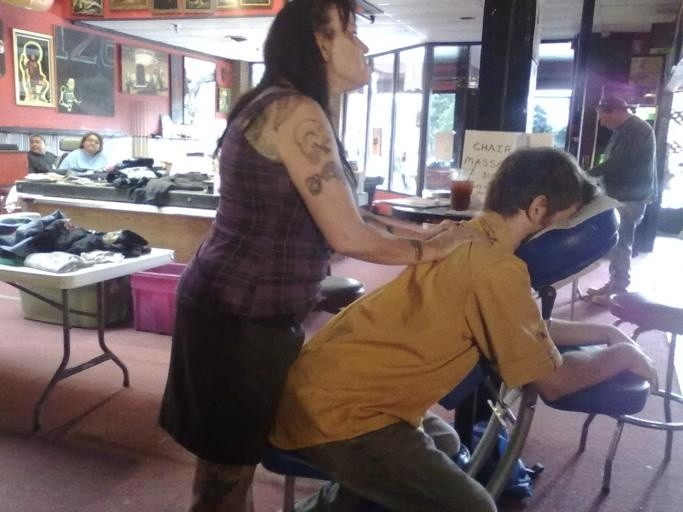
[12,29,54,110]
[69,0,272,18]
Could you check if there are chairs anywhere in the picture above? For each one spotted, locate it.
[259,195,650,512]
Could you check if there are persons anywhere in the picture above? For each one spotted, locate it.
[55,129,107,174]
[24,134,69,174]
[582,94,661,309]
[266,143,661,510]
[155,0,501,511]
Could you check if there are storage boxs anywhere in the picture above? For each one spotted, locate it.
[19,263,188,336]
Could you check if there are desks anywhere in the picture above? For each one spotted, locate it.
[1,212,173,431]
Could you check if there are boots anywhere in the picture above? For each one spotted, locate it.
[586,276,631,306]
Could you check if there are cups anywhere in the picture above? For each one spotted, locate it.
[448,171,474,211]
